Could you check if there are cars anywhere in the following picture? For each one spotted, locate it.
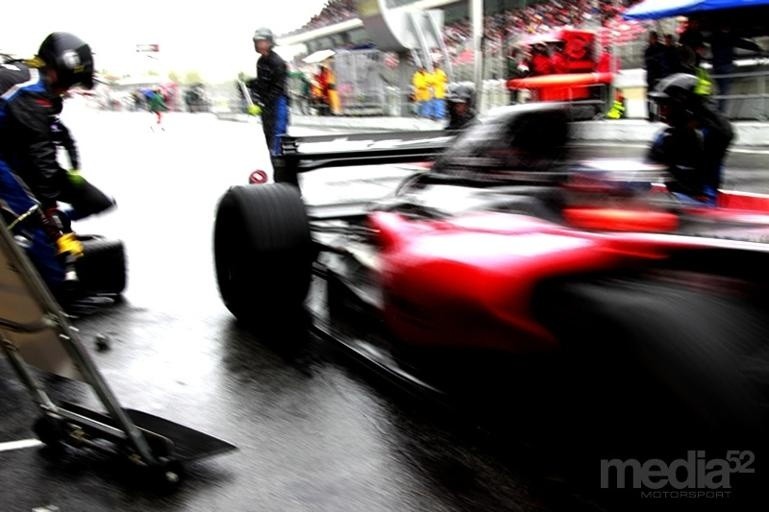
[213,101,769,511]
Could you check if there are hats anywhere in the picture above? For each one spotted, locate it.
[252,28,274,40]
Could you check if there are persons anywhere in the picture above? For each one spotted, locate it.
[429,60,447,120]
[646,31,726,123]
[428,81,483,170]
[643,73,737,208]
[236,27,302,198]
[412,65,434,119]
[0,31,111,332]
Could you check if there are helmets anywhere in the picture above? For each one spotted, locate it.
[646,74,709,101]
[34,32,94,82]
